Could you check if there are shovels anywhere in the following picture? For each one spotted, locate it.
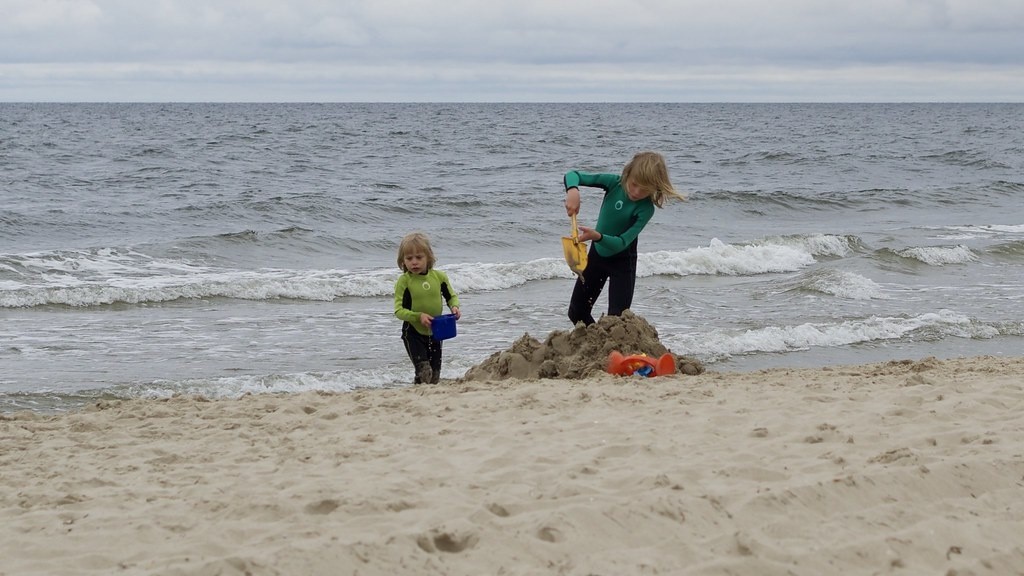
[561,211,589,275]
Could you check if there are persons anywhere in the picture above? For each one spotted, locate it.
[564,151,686,330]
[394,232,461,385]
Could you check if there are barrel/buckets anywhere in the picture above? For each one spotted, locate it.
[431,314,457,340]
